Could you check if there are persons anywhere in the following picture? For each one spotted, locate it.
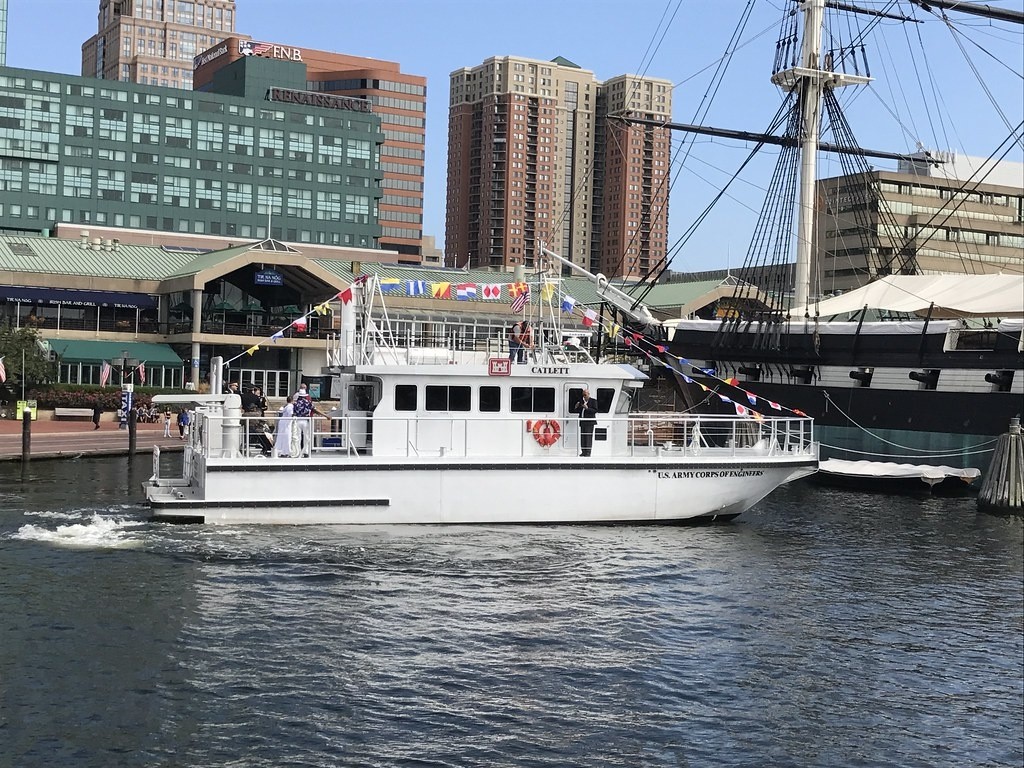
[574,390,599,457]
[225,379,329,459]
[135,399,189,440]
[91,401,103,430]
[508,320,530,365]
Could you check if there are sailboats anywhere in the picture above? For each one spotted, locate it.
[601,1,1023,485]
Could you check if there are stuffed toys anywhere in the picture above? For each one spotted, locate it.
[96,358,148,389]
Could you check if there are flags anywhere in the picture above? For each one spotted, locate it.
[379,277,599,328]
[733,390,783,419]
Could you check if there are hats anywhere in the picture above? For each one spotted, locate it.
[298,389,309,396]
[228,380,237,384]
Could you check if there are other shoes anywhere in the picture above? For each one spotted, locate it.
[304,454,309,458]
[579,453,585,456]
[95,426,100,430]
[163,435,166,438]
[168,435,171,438]
[585,453,590,457]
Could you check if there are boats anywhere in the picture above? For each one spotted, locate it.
[812,454,984,491]
[139,238,822,527]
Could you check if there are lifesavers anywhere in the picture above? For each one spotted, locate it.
[531,419,563,448]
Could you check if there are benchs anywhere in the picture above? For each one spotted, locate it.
[54,408,94,422]
